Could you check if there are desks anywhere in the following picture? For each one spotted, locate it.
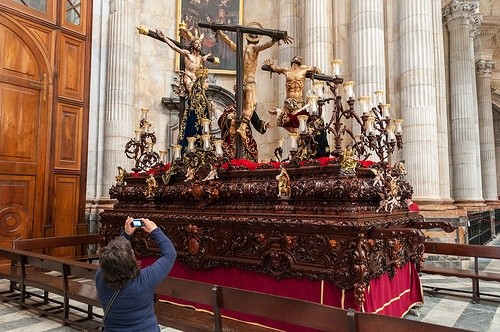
[96,210,424,332]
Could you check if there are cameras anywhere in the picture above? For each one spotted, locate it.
[130,219,145,228]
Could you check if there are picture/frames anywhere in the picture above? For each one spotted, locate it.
[175,0,244,75]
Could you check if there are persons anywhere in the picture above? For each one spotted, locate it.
[155,28,213,97]
[176,73,211,159]
[371,160,408,213]
[340,144,357,169]
[210,23,295,140]
[145,173,158,198]
[218,90,275,163]
[184,163,195,182]
[276,162,290,197]
[263,56,340,134]
[115,166,126,185]
[202,167,220,182]
[95,215,178,332]
[403,197,419,271]
